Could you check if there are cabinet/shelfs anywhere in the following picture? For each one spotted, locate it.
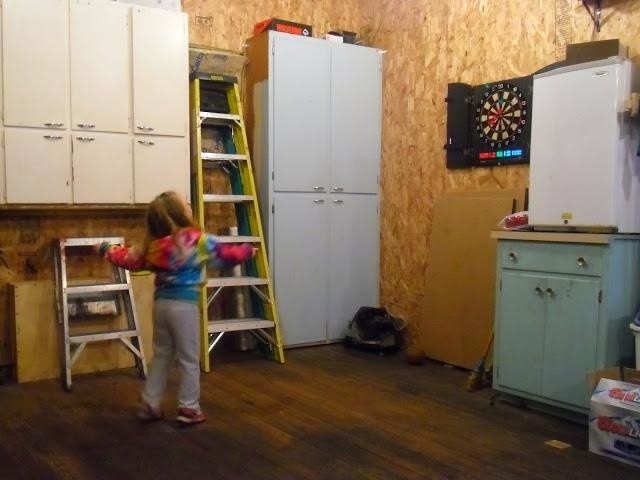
[247,30,385,350]
[491,230,640,425]
[0,0,191,213]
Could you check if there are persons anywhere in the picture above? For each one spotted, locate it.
[92,191,261,426]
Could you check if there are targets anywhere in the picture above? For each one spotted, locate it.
[473,82,527,149]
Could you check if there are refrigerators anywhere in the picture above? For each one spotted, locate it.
[527,55,639,233]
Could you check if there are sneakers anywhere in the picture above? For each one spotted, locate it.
[177,406,208,425]
[135,397,166,420]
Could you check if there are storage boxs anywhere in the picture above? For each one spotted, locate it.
[587,366,639,469]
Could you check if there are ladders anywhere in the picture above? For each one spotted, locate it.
[189,70,285,373]
[52,232,152,388]
